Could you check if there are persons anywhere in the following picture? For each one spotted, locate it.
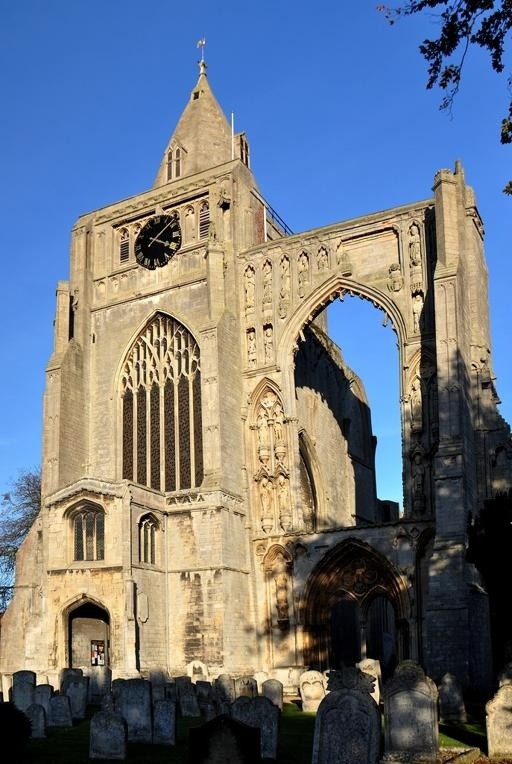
[97,642,105,665]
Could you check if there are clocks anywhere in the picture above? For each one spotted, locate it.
[134,214,181,270]
